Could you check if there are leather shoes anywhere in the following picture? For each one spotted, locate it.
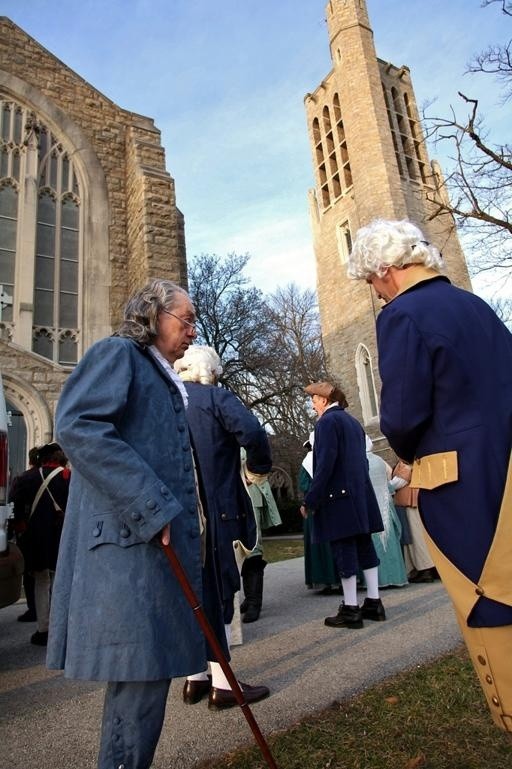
[183,674,212,704]
[410,570,433,583]
[325,600,363,629]
[209,682,269,711]
[359,597,385,621]
[30,630,47,646]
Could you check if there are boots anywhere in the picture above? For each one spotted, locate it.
[242,560,266,622]
[18,593,36,621]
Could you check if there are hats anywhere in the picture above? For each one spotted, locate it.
[40,443,68,466]
[304,382,348,408]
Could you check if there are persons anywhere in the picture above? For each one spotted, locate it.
[389,458,441,583]
[342,220,511,736]
[236,446,282,624]
[4,442,71,644]
[44,278,224,768]
[364,432,409,588]
[299,429,343,595]
[303,381,386,629]
[171,344,273,712]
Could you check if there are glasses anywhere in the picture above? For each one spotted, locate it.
[160,309,195,334]
